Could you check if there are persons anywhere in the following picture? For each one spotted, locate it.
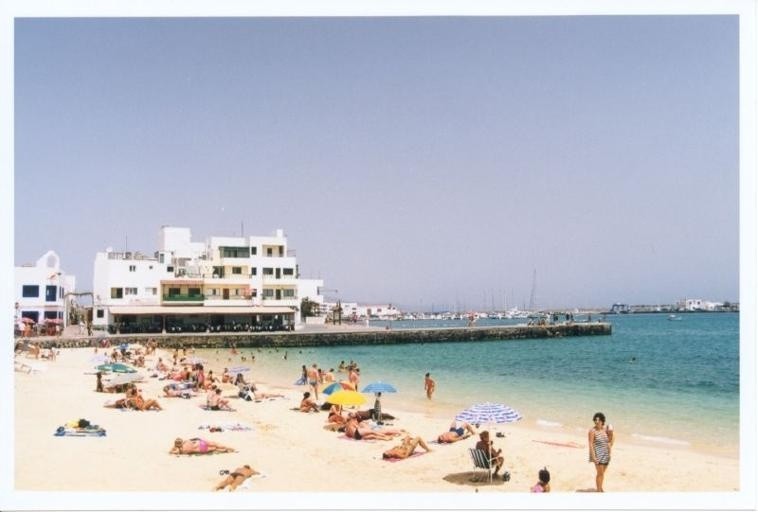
[214,464,260,491]
[24,323,30,336]
[588,411,615,492]
[56,325,60,336]
[96,339,293,411]
[530,466,552,493]
[382,435,431,459]
[57,419,106,436]
[424,372,436,399]
[474,430,503,479]
[300,361,408,443]
[169,437,236,454]
[41,348,59,361]
[437,421,474,444]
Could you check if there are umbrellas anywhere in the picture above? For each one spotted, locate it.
[455,400,523,483]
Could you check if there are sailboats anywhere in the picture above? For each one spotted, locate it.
[396,289,550,320]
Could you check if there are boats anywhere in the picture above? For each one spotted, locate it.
[601,298,730,313]
[668,313,681,321]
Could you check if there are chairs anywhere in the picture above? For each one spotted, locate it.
[469,448,500,484]
[237,382,257,402]
